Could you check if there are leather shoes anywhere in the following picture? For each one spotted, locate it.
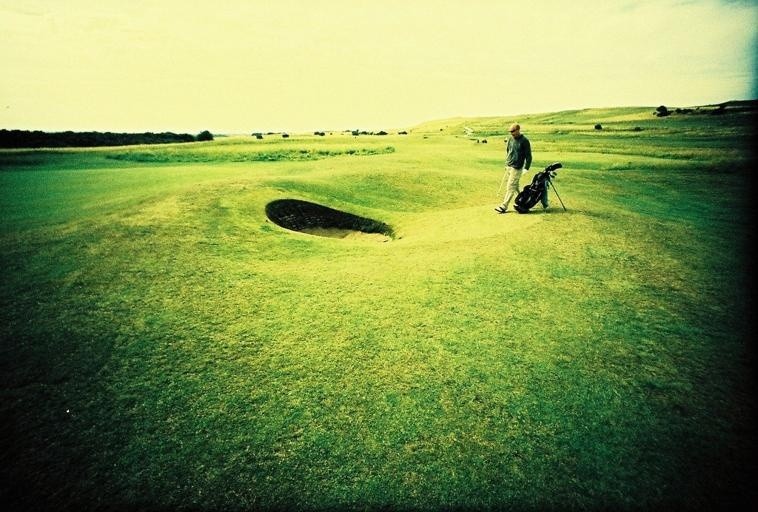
[495,207,505,212]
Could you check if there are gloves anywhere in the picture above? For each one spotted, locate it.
[523,168,527,173]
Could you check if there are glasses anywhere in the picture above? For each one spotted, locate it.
[511,129,517,132]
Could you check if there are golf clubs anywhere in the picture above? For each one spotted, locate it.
[544,161,562,178]
[496,167,508,197]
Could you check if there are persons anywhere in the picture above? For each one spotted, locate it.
[495,123,532,213]
